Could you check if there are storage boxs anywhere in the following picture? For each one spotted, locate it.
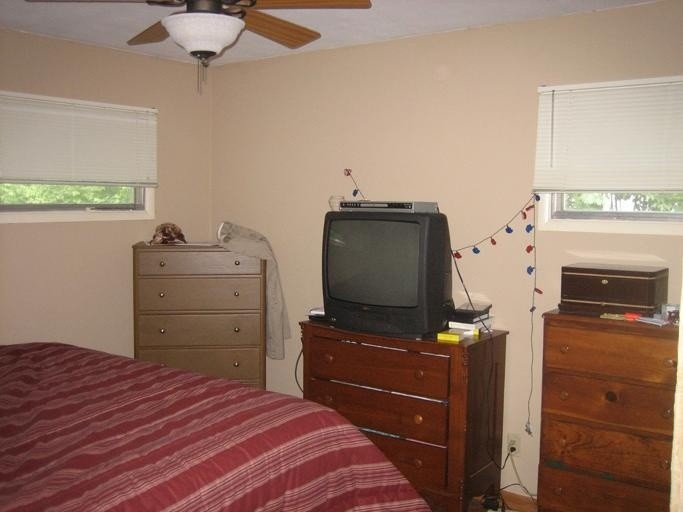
[558,262,669,318]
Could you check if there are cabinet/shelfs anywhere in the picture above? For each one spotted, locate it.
[297,320,509,512]
[537,308,678,512]
[132,241,266,390]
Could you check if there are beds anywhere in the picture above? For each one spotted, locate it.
[0,342,431,510]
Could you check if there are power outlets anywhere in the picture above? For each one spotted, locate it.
[507,433,521,457]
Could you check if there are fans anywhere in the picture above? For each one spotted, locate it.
[126,0,372,49]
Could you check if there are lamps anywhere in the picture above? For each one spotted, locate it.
[147,0,257,67]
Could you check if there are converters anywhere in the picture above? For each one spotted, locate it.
[484,499,499,510]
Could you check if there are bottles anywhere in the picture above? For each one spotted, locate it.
[329,195,345,211]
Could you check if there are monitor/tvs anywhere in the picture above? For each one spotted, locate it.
[322,211,453,339]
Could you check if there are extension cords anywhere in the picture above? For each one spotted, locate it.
[486,497,503,512]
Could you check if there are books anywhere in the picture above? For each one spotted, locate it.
[309,306,326,323]
[436,301,494,342]
